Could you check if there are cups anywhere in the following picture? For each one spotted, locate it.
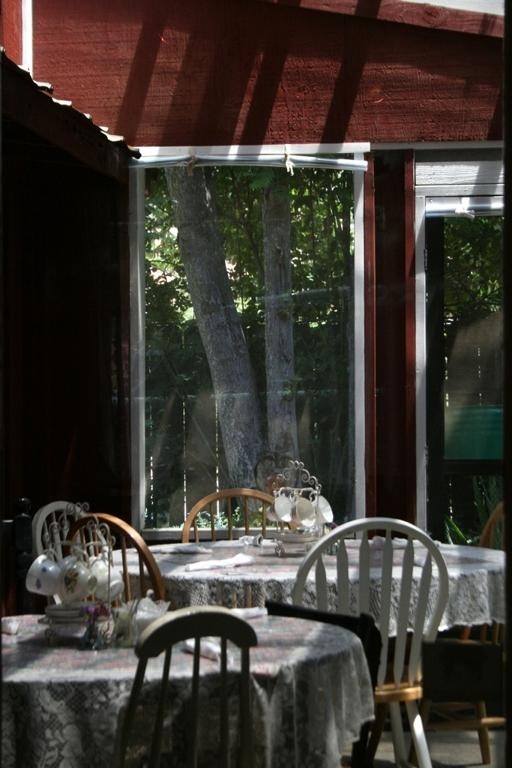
[266,493,334,530]
[28,553,126,605]
[111,599,171,647]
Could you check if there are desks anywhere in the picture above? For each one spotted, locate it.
[1,610,376,766]
[99,535,508,640]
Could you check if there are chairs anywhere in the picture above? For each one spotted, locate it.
[291,508,511,767]
[30,501,166,605]
[109,605,258,766]
[181,488,292,543]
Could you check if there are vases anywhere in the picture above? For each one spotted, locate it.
[111,606,141,649]
